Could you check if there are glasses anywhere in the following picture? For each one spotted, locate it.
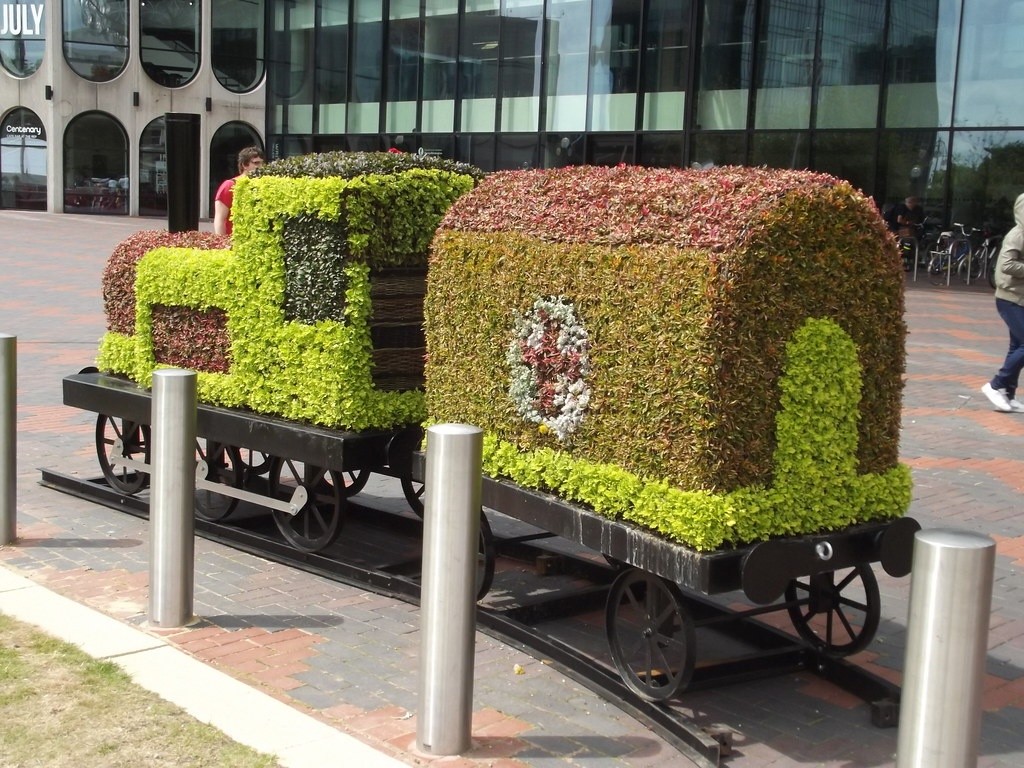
[250,160,261,165]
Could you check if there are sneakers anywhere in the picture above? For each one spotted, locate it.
[982,382,1024,413]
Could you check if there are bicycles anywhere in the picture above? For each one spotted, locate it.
[897,212,1003,289]
[92,188,125,210]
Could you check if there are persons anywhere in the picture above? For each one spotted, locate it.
[213,144,265,237]
[887,195,918,241]
[981,192,1024,413]
